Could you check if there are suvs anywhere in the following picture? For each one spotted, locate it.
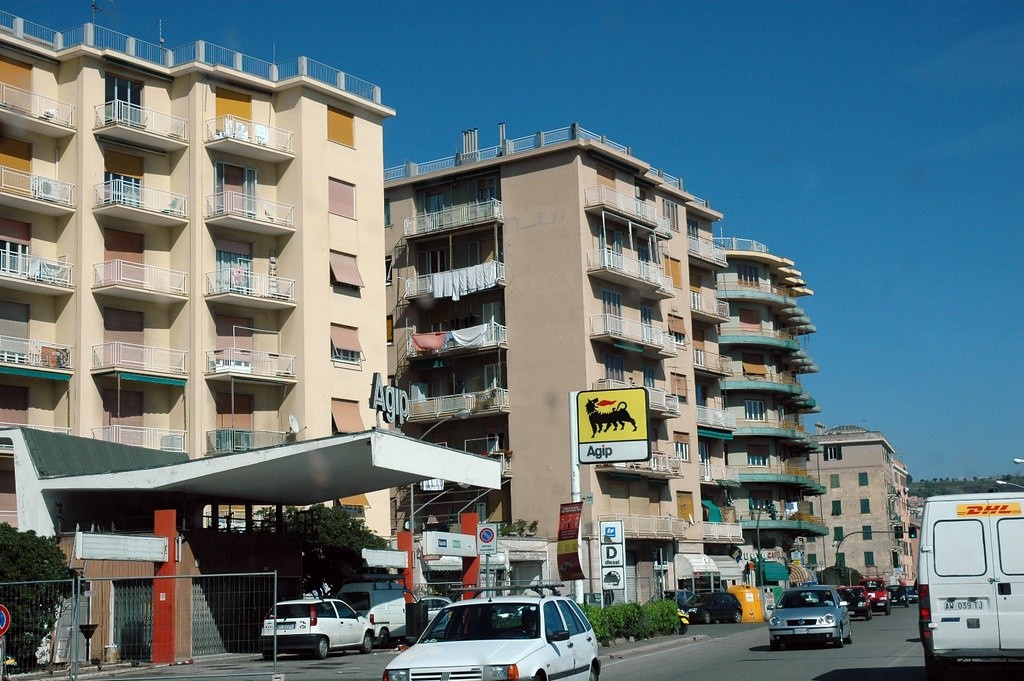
[649,590,694,611]
[257,599,377,658]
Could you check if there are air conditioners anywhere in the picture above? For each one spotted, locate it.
[37,179,57,198]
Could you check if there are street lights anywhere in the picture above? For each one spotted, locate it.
[411,408,470,543]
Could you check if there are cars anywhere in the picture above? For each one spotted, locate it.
[858,578,891,615]
[767,586,853,648]
[382,593,601,681]
[418,597,453,632]
[836,586,872,621]
[904,586,918,604]
[681,592,744,624]
[886,585,909,608]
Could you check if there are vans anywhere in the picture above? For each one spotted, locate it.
[334,582,406,649]
[917,493,1024,681]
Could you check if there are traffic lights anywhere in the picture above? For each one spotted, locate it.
[909,526,917,538]
